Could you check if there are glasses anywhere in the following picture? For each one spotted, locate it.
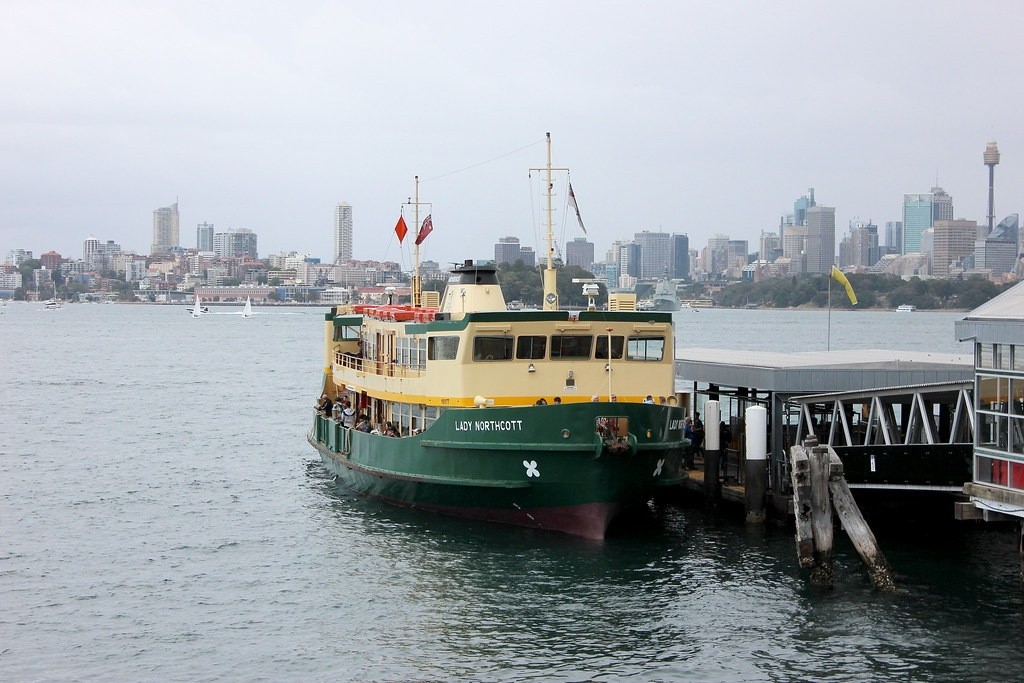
[612,396,617,399]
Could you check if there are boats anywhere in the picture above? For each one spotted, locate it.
[186,306,209,315]
[45,280,60,309]
[307,132,693,542]
[896,304,917,312]
[635,279,682,311]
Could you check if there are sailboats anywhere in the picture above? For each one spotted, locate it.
[241,295,253,319]
[193,294,201,318]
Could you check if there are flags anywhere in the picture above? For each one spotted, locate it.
[395,218,408,243]
[568,183,587,234]
[415,215,434,245]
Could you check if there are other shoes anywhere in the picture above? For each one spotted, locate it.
[685,466,698,471]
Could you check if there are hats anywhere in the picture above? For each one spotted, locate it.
[322,394,328,399]
[412,428,421,433]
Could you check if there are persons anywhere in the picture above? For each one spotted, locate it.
[535,397,561,405]
[314,394,401,438]
[683,413,703,471]
[644,395,667,406]
[591,394,620,435]
